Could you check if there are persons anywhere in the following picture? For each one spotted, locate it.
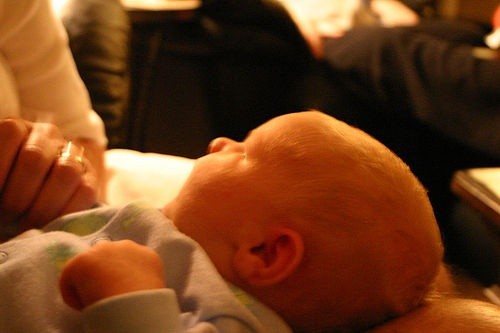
[0,111,443,333]
[0,0,107,241]
